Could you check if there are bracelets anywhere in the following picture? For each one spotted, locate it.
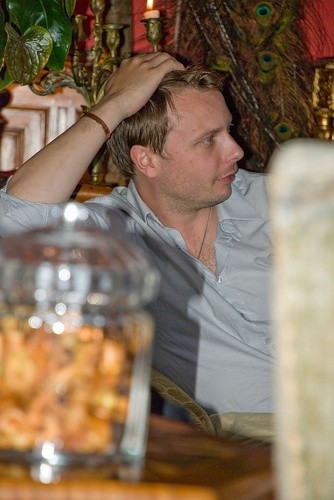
[80,112,111,141]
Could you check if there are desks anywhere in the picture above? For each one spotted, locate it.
[1,412,271,499]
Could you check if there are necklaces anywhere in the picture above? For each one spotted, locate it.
[197,205,213,260]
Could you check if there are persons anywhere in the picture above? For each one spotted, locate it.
[0,52,277,417]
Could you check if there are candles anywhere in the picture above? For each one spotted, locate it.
[143,0,159,19]
